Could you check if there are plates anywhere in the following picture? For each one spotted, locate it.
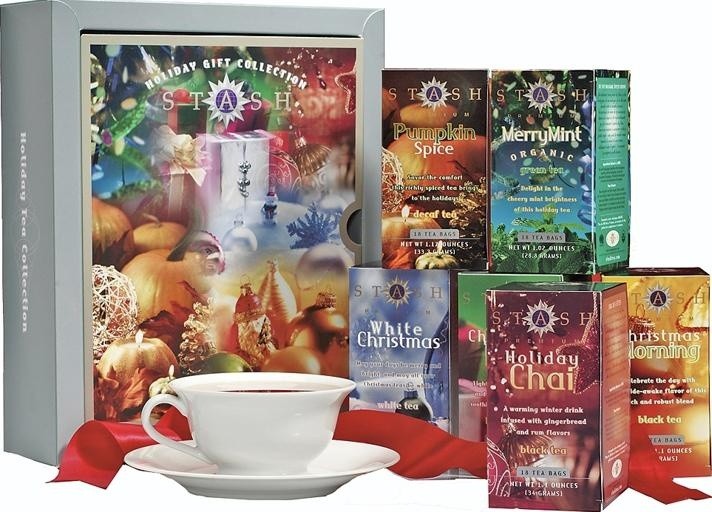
[124,439,400,500]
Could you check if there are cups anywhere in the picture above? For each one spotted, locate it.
[141,372,357,474]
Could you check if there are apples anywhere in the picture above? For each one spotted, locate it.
[201,352,253,375]
[260,346,329,374]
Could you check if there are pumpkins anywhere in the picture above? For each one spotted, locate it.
[92,198,210,379]
[382,102,486,270]
